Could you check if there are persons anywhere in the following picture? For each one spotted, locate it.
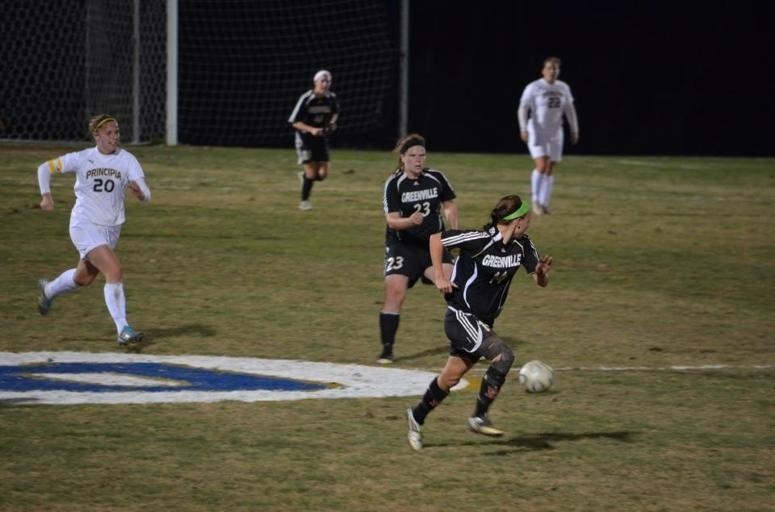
[516,57,581,216]
[285,70,341,210]
[34,111,153,346]
[378,133,458,361]
[402,195,554,452]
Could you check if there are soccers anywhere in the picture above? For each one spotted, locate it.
[518,360,554,392]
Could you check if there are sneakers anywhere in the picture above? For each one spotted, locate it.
[299,172,312,211]
[406,408,421,452]
[468,417,504,436]
[532,204,544,215]
[38,279,51,317]
[118,326,144,345]
[377,355,393,364]
